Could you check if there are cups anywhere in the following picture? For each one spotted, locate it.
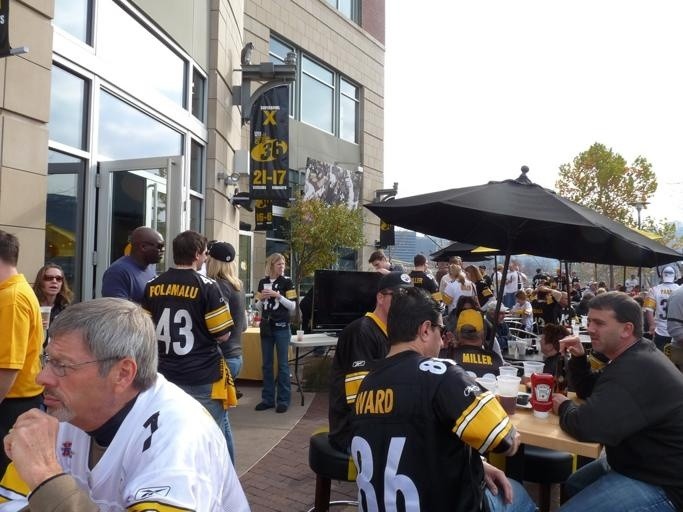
[498,366,517,377]
[497,376,520,415]
[264,284,271,290]
[478,379,496,391]
[296,330,303,340]
[40,307,50,329]
[523,361,544,386]
[572,324,579,336]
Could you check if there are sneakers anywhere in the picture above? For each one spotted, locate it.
[275,405,287,413]
[236,391,244,399]
[255,402,275,410]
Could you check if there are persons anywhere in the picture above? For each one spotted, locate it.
[662,283,682,369]
[551,291,578,325]
[614,275,652,339]
[539,325,578,390]
[298,280,314,335]
[645,267,682,351]
[438,256,465,295]
[252,253,297,413]
[102,227,165,302]
[141,230,233,438]
[569,291,596,319]
[328,271,415,458]
[0,230,45,482]
[466,265,495,311]
[0,297,249,511]
[442,264,481,315]
[533,269,609,298]
[443,296,501,358]
[369,251,406,275]
[408,254,442,301]
[510,290,533,337]
[439,308,502,385]
[479,265,492,286]
[493,264,509,300]
[31,265,75,347]
[506,264,524,309]
[349,288,539,512]
[552,291,682,512]
[507,262,527,291]
[205,240,249,466]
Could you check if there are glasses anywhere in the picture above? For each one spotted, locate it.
[38,351,123,377]
[432,322,448,338]
[381,292,394,295]
[44,274,63,282]
[144,241,165,250]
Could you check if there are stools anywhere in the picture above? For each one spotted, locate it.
[305,427,360,512]
[506,443,578,512]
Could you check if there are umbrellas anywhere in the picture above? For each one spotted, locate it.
[429,256,494,262]
[429,242,526,300]
[362,166,682,352]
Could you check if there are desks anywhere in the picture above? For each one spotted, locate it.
[237,327,292,384]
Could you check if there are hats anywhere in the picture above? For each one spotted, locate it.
[456,308,484,334]
[210,242,235,263]
[489,300,508,312]
[377,271,415,292]
[661,266,676,284]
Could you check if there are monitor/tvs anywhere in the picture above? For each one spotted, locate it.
[312,271,403,333]
